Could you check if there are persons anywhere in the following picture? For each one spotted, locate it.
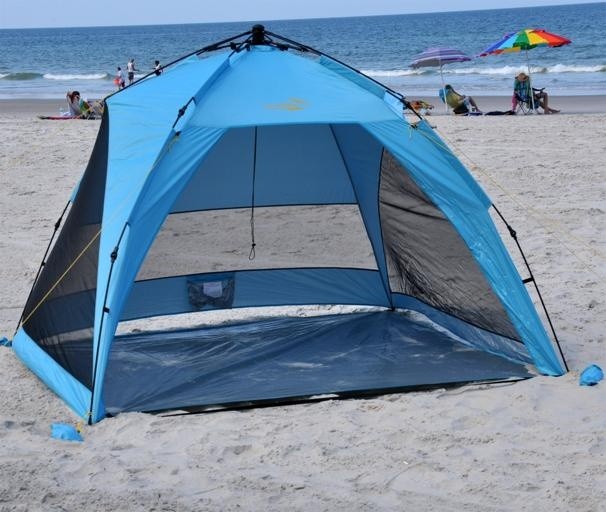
[116,67,125,90]
[68,90,104,120]
[127,59,139,85]
[529,90,561,115]
[440,84,486,115]
[153,61,162,72]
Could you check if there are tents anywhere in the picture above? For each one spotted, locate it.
[10,24,573,425]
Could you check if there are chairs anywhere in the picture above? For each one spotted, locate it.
[66,93,104,119]
[439,87,472,117]
[513,83,550,116]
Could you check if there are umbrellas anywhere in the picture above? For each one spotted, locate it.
[476,28,572,111]
[408,47,471,112]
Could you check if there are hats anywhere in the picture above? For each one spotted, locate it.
[517,73,528,82]
